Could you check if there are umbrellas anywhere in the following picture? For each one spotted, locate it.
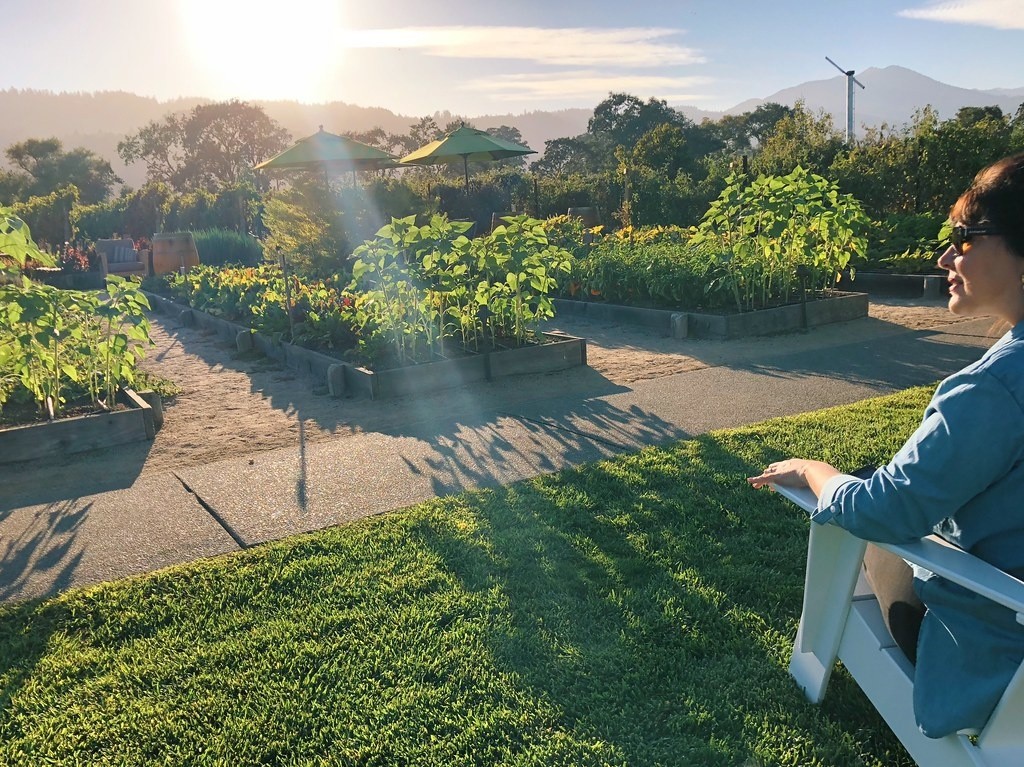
[395,121,539,197]
[249,123,419,206]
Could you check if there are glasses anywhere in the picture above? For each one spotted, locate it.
[951,225,1004,255]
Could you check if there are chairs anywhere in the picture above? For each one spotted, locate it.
[769,481,1024,767]
[93,239,149,287]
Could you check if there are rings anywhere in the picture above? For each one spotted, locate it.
[770,468,773,473]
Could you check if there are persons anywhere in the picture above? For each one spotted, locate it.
[747,148,1024,738]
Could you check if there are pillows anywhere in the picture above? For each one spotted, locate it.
[111,246,138,263]
